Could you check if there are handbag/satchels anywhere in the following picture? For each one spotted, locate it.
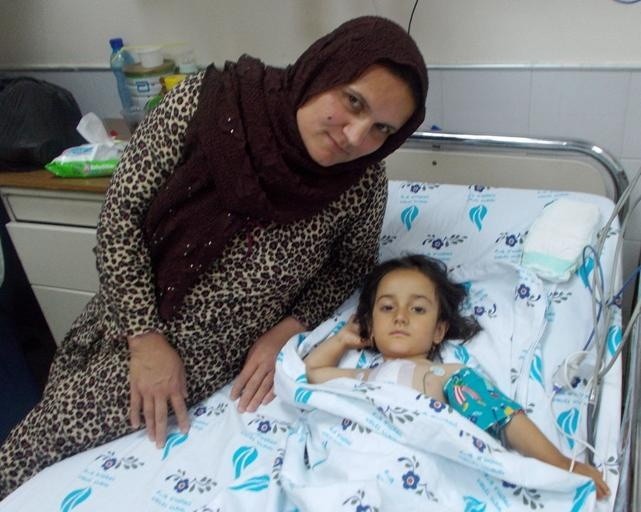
[1,77,89,172]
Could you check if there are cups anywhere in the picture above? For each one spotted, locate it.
[136,48,164,68]
[120,107,146,137]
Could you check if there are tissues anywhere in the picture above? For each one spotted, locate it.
[45,112,128,179]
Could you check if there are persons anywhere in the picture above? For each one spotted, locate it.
[0,16,428,503]
[300,251,611,503]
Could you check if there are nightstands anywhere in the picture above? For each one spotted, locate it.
[0,116,131,348]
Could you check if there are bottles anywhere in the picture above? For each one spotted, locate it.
[109,38,135,110]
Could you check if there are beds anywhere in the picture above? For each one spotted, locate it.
[0,130,640,512]
